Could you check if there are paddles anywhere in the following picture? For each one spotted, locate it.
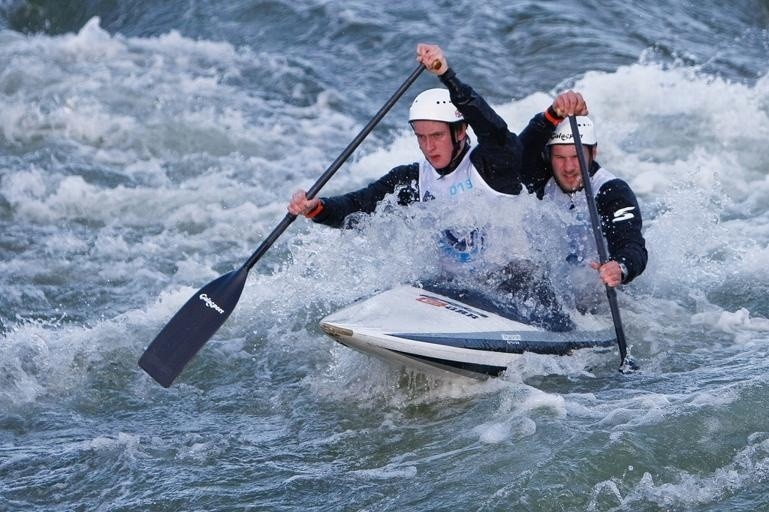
[138,60,441,388]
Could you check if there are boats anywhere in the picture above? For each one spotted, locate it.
[318,272,619,386]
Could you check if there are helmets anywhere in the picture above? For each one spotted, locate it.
[407,88,465,123]
[548,114,597,145]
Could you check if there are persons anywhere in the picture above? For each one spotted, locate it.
[518,91,649,286]
[288,41,527,272]
